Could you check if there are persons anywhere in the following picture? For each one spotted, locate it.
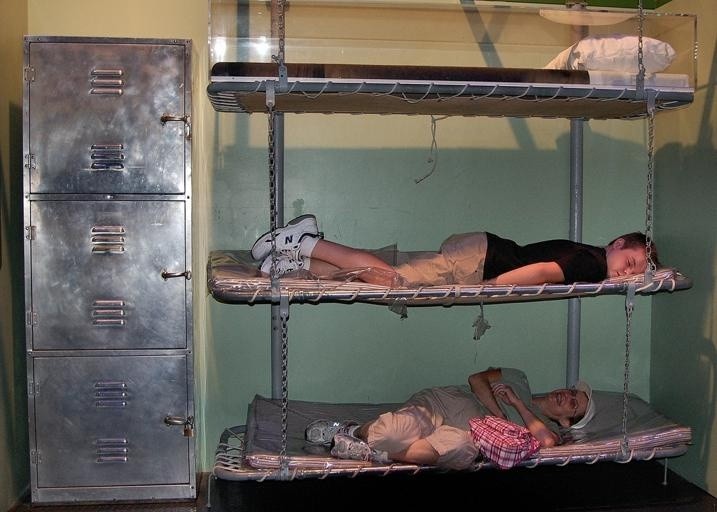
[304,366,596,470]
[250,213,658,289]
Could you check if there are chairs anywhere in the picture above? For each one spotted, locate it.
[304,418,358,447]
[251,214,318,260]
[258,254,303,276]
[330,434,371,461]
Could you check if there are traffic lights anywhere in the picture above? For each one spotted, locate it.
[544,34,675,73]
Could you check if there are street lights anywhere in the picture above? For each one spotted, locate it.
[468,415,539,469]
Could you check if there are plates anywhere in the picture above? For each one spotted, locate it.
[23,37,198,502]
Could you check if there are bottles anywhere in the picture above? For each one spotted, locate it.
[571,381,596,430]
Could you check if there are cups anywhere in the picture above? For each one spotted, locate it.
[569,384,579,409]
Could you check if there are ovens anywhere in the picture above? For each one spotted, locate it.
[205,0,692,510]
